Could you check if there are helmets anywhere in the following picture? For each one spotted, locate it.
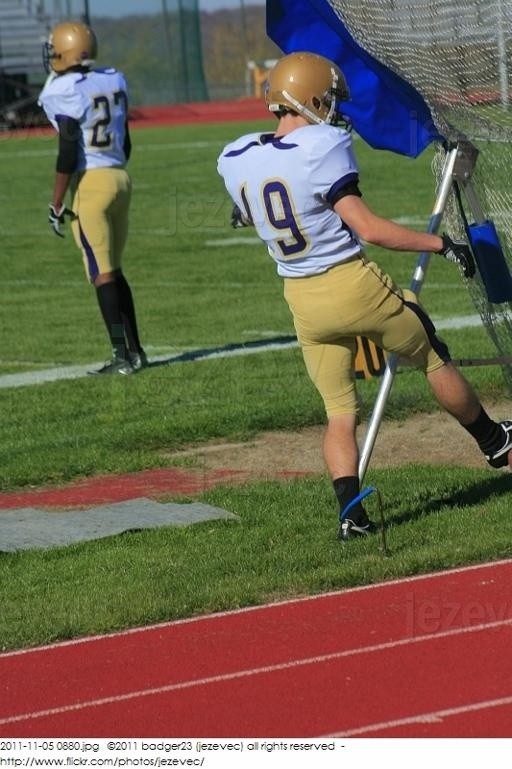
[264,50,352,127]
[48,21,98,74]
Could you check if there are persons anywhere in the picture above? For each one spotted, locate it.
[35,20,148,375]
[215,52,512,541]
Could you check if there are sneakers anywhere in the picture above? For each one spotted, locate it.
[484,419,511,470]
[127,348,148,372]
[87,351,134,376]
[337,519,378,541]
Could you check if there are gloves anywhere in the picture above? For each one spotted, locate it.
[48,203,75,238]
[438,234,476,280]
[230,203,250,229]
